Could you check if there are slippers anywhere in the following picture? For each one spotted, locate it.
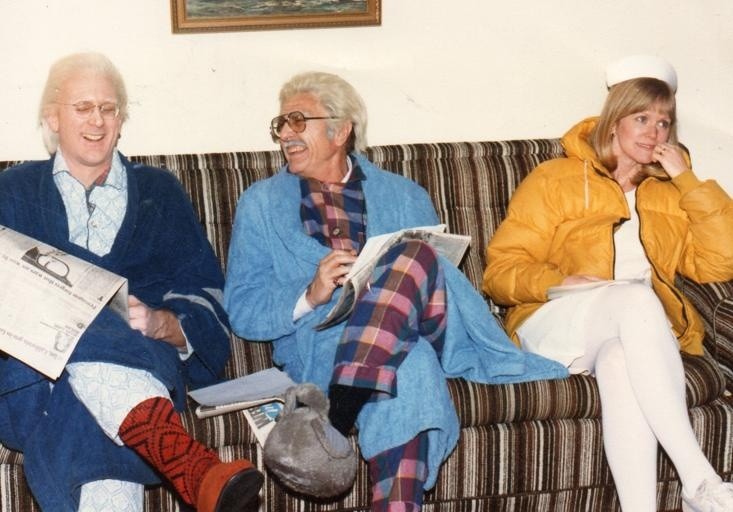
[263,382,358,497]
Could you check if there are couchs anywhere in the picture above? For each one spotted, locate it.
[2,136,731,512]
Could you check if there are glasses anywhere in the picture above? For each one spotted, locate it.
[270,111,338,138]
[49,100,120,119]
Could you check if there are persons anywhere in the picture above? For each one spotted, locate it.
[481,54,732,512]
[221,69,481,511]
[1,52,264,510]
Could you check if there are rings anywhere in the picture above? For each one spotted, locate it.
[660,148,666,154]
[333,277,340,286]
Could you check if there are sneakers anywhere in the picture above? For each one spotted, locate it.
[681,476,733,512]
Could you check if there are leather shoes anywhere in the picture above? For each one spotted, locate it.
[196,460,264,512]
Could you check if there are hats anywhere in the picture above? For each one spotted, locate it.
[604,57,678,93]
